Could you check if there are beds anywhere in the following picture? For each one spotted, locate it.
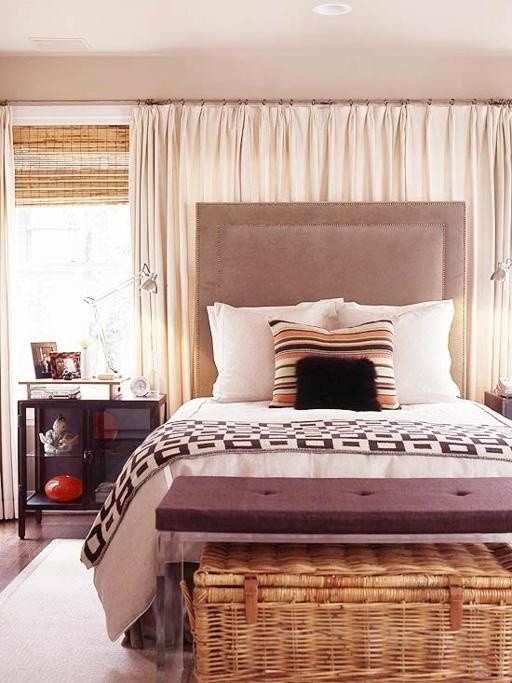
[200,296,345,402]
[338,297,462,406]
[266,315,403,411]
[80,200,511,681]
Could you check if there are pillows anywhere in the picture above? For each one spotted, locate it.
[293,350,383,414]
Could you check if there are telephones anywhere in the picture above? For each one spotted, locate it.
[496,377,512,398]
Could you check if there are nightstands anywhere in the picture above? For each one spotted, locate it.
[16,391,168,540]
[481,390,512,417]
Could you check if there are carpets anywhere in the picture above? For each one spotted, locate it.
[0,538,185,681]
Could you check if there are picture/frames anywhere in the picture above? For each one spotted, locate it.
[49,350,82,379]
[31,341,59,379]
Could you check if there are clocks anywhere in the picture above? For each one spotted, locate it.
[130,375,151,396]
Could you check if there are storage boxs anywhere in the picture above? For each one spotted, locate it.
[93,480,115,502]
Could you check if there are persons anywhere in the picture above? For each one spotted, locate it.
[43,416,72,454]
[40,353,52,374]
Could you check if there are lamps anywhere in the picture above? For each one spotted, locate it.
[487,254,512,284]
[82,262,160,379]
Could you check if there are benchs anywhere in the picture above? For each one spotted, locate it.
[149,471,512,681]
